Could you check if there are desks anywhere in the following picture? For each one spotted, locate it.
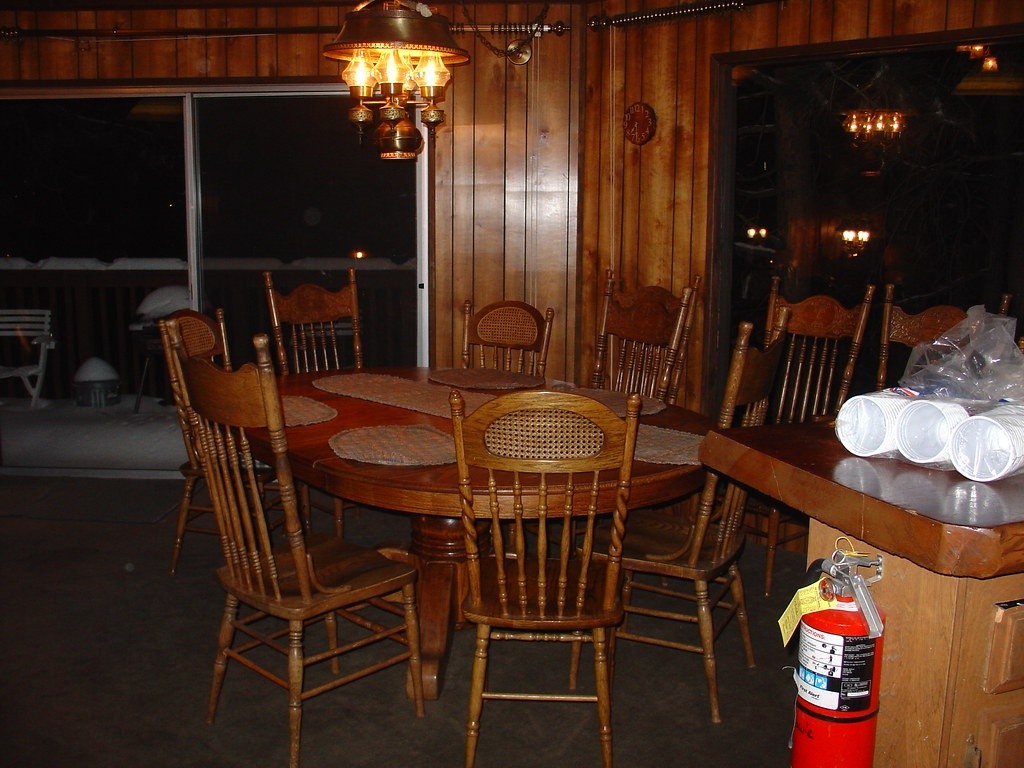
[703,421,1023,768]
[236,364,714,696]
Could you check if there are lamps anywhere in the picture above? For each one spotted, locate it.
[321,0,472,158]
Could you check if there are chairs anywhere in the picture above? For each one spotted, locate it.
[130,284,189,412]
[168,267,1017,767]
[0,311,57,408]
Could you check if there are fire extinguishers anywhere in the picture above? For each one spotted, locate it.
[777,536,886,768]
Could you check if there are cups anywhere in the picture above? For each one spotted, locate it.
[837,389,1024,482]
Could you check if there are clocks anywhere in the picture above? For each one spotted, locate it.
[623,102,656,145]
